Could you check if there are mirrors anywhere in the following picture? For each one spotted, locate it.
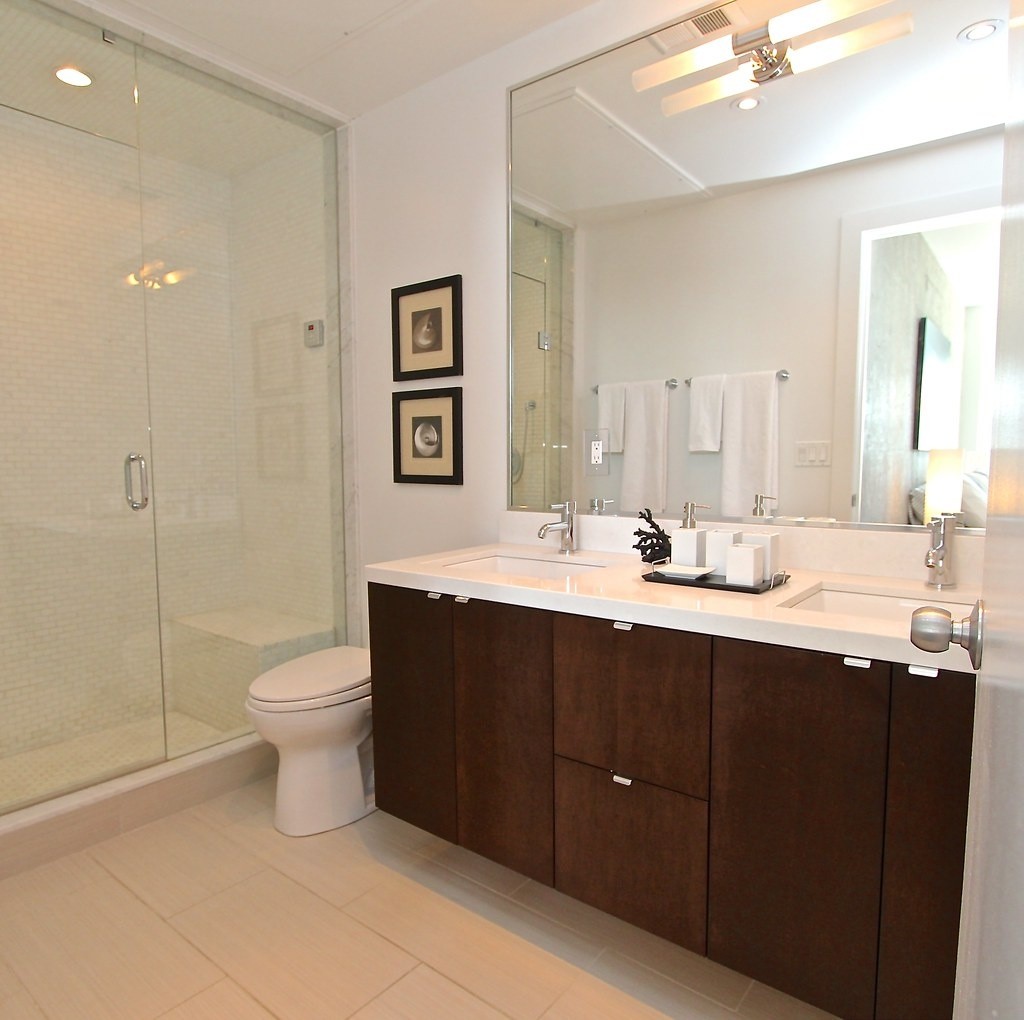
[502,0,1024,540]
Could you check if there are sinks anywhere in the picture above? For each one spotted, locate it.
[442,551,611,589]
[775,577,981,647]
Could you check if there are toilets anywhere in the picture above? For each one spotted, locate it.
[241,644,387,839]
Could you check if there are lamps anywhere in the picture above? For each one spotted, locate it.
[957,18,1006,44]
[127,259,187,289]
[731,95,763,113]
[53,65,95,90]
[631,0,890,93]
[660,13,912,116]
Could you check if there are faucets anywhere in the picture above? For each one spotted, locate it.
[926,514,959,590]
[537,500,580,556]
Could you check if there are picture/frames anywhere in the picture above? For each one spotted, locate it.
[391,274,463,381]
[391,387,464,487]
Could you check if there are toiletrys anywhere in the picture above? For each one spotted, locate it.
[670,500,712,569]
[743,493,778,523]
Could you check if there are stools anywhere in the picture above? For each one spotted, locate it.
[178,609,335,730]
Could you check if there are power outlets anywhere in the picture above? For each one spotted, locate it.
[304,320,324,348]
[590,441,602,464]
[793,440,831,467]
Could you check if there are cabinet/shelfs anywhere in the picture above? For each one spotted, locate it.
[552,755,711,956]
[713,635,976,1019]
[369,581,556,887]
[552,611,713,802]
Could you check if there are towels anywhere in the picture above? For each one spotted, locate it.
[618,378,671,513]
[719,370,780,519]
[687,372,727,454]
[596,384,625,454]
